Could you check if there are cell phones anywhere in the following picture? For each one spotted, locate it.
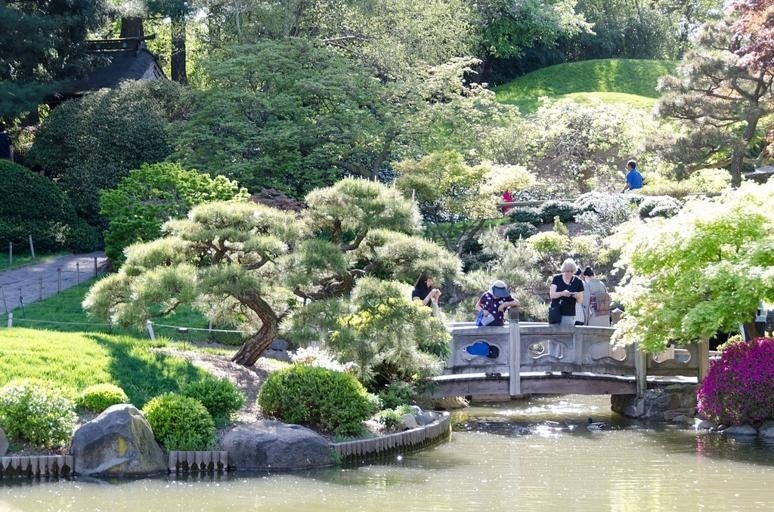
[568,292,575,295]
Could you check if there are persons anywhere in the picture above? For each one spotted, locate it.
[502,190,514,213]
[411,271,440,316]
[579,264,615,326]
[567,269,587,374]
[547,257,583,376]
[619,159,643,194]
[474,279,522,378]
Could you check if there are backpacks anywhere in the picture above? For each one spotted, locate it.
[590,283,610,316]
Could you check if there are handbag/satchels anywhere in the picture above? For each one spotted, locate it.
[432,302,439,314]
[476,309,495,326]
[548,304,561,324]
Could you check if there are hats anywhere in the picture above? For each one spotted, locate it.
[491,280,510,297]
